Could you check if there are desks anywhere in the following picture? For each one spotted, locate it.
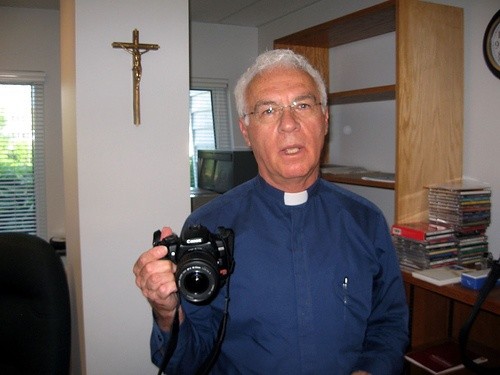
[397,264,500,375]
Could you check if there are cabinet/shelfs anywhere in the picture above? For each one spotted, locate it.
[190,187,223,214]
[274,1,464,238]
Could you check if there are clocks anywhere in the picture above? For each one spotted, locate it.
[483,9,500,82]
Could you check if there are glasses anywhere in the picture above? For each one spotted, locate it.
[242,99,325,122]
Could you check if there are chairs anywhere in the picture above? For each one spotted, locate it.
[0,233,73,375]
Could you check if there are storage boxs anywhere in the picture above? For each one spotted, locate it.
[462,269,492,291]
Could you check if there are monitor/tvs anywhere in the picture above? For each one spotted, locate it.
[212,158,234,193]
[198,157,216,190]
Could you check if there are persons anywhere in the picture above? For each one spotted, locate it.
[120,44,149,89]
[134,48,410,375]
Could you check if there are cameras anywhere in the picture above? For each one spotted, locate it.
[150,223,236,303]
